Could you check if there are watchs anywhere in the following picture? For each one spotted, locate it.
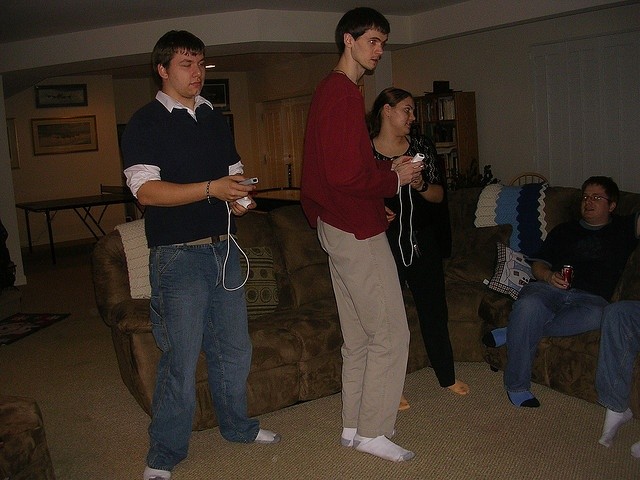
[417,182,428,192]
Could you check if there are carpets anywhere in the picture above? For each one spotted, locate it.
[0,312,62,346]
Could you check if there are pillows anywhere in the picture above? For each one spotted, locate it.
[485,241,537,299]
[240,246,279,320]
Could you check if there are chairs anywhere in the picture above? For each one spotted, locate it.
[510,172,550,188]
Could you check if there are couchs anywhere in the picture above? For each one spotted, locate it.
[0,394,56,480]
[90,187,640,431]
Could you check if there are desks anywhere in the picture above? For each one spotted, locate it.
[15,183,146,261]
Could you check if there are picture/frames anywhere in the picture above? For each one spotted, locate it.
[200,78,230,111]
[6,118,22,169]
[34,84,88,108]
[31,115,98,155]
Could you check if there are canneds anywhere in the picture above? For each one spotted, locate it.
[561,264,575,288]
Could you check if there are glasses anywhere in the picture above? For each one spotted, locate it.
[581,195,607,202]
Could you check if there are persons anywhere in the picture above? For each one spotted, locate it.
[299,7,425,463]
[119,30,282,480]
[594,300,640,458]
[365,87,472,412]
[482,176,640,408]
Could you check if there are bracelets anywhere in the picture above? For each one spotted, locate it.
[206,178,215,205]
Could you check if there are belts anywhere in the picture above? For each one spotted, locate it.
[175,233,238,248]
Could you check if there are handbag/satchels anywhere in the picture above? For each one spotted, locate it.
[395,215,443,290]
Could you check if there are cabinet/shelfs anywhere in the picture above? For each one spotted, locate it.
[409,92,480,187]
